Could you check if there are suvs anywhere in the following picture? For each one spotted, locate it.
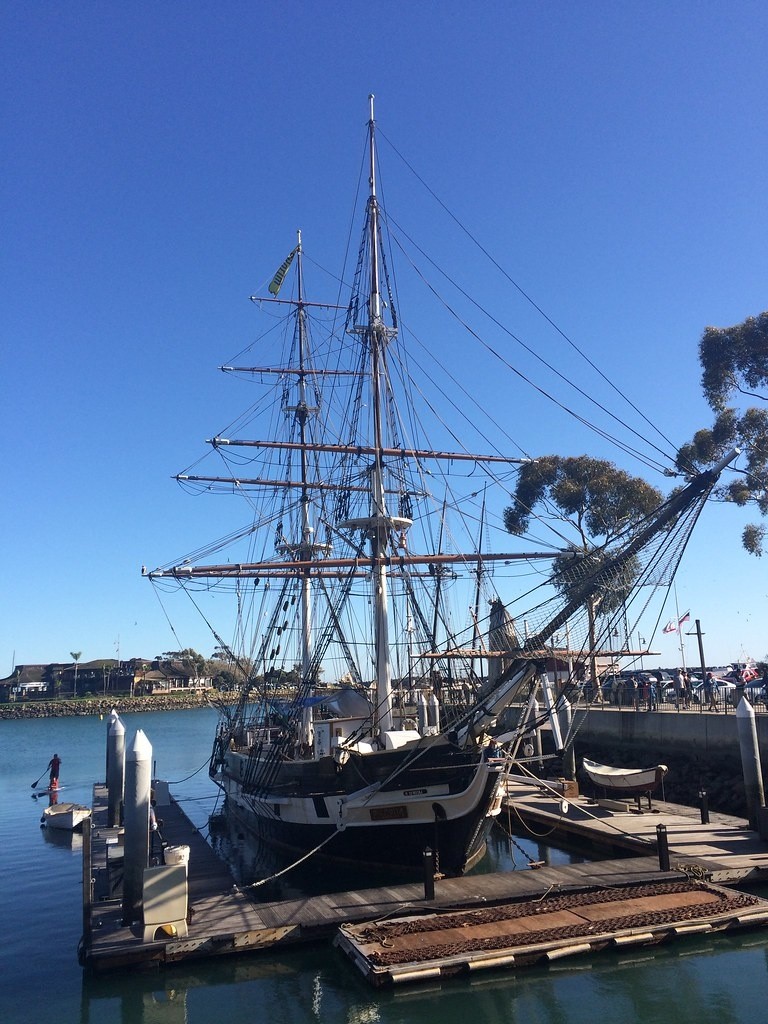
[722,669,757,685]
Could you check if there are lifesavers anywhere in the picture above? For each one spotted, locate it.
[400,718,419,733]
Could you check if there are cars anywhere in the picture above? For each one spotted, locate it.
[726,677,766,704]
[666,679,737,703]
[579,670,715,702]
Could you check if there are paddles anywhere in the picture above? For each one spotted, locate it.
[31,765,52,789]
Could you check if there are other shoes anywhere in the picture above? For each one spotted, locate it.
[709,708,712,712]
[682,706,686,709]
[653,709,656,711]
[648,709,651,711]
[716,709,719,712]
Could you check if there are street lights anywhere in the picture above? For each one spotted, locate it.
[551,634,562,700]
[638,638,648,671]
[608,628,620,679]
[446,633,456,692]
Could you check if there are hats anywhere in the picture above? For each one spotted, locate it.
[677,668,682,671]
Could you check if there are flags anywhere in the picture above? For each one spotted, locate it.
[662,621,676,634]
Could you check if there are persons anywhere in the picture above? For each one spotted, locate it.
[642,681,656,711]
[733,668,746,691]
[462,680,470,703]
[47,754,62,782]
[706,673,720,713]
[626,676,637,706]
[760,667,768,697]
[673,669,686,709]
[684,672,694,708]
[538,763,547,780]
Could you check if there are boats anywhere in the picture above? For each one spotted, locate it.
[43,800,93,831]
[580,754,669,798]
[43,826,84,850]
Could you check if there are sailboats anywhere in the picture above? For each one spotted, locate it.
[141,90,740,890]
